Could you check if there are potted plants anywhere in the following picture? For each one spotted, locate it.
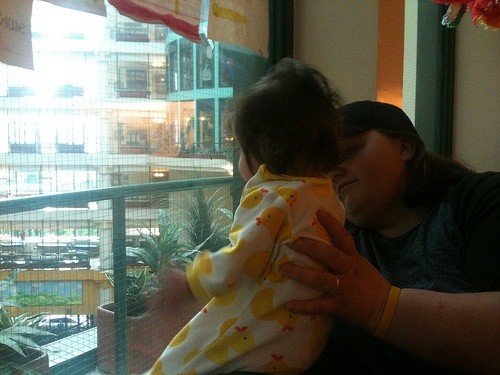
[0,304,59,375]
[96,163,234,375]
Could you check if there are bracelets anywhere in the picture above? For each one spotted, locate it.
[373,285,401,339]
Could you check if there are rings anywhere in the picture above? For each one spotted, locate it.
[331,275,340,294]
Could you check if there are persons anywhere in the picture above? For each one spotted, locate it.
[281,100,500,375]
[147,57,347,375]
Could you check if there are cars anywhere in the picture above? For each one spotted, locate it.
[38,316,86,331]
